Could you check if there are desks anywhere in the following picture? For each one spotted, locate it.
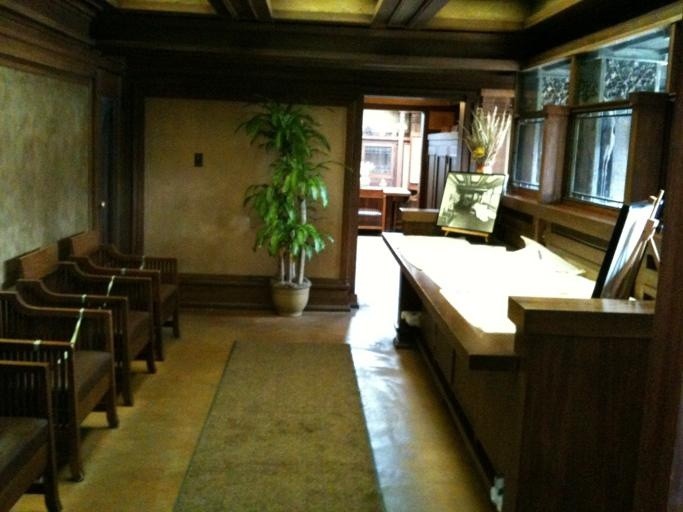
[381,187,411,231]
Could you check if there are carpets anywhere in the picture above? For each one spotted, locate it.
[173,338,385,511]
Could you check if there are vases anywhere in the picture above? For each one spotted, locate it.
[474,162,492,174]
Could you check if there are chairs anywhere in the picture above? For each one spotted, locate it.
[13,243,155,404]
[357,188,386,233]
[0,359,65,510]
[1,285,120,479]
[61,232,179,361]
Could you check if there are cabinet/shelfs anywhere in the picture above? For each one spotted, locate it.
[382,230,617,511]
[426,132,458,208]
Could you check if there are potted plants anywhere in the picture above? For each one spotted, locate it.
[231,100,354,319]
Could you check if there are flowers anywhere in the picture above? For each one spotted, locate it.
[457,105,512,165]
